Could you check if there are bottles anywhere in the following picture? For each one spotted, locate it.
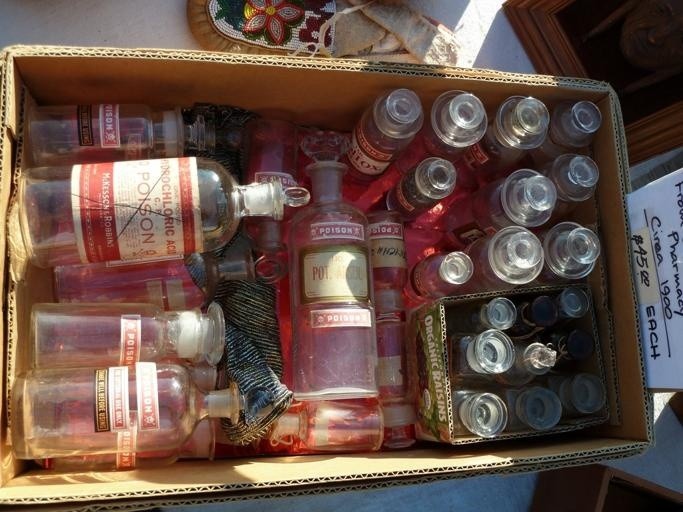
[16,88,610,466]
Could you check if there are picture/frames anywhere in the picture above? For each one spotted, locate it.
[502,1,682,169]
